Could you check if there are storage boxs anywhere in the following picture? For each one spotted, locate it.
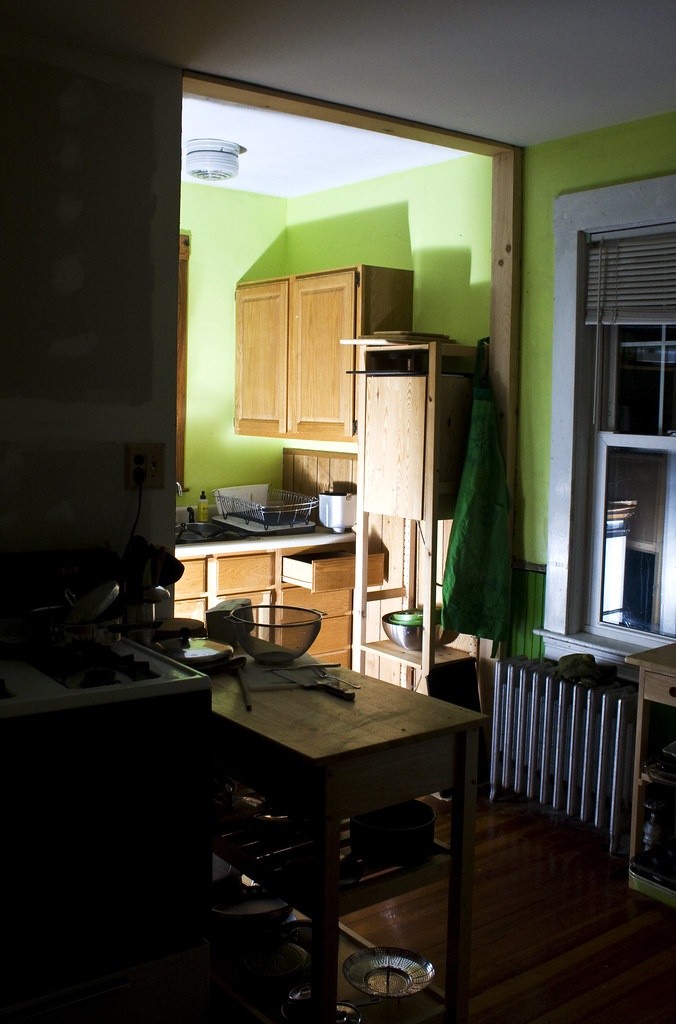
[319,493,357,534]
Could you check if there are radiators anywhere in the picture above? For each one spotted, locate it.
[490,656,640,854]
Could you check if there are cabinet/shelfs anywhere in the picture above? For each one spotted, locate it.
[338,338,487,697]
[234,265,413,452]
[173,543,384,676]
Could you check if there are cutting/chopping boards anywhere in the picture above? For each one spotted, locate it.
[232,644,339,692]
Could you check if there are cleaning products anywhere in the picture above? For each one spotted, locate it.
[197,490,209,522]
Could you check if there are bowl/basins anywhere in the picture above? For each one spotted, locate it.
[350,799,437,869]
[175,522,224,540]
[382,609,444,651]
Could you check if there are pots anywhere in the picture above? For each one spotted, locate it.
[48,580,163,653]
[318,492,357,533]
[211,892,292,928]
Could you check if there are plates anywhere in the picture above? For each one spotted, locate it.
[343,946,435,997]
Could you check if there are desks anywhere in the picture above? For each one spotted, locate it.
[625,644,675,908]
[204,665,491,1024]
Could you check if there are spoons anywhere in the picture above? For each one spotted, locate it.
[312,666,361,690]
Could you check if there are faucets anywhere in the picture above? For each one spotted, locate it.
[176,482,183,496]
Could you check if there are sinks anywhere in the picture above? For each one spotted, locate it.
[174,521,253,544]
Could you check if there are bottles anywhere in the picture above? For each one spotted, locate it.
[197,491,209,523]
[643,813,660,851]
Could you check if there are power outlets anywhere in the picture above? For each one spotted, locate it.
[126,442,167,489]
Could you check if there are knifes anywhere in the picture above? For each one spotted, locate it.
[273,668,355,700]
